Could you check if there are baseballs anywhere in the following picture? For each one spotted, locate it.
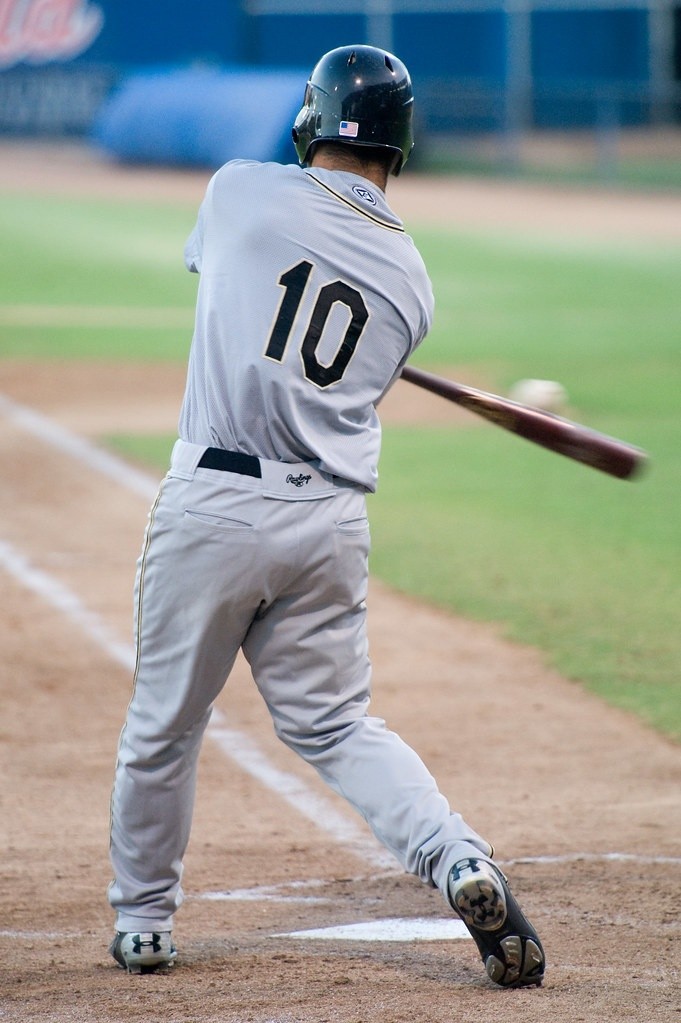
[514,378,562,409]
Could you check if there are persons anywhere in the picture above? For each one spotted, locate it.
[105,45,547,986]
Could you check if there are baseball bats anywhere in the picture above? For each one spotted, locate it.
[400,365,646,483]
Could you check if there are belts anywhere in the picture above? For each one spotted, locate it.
[196,447,262,481]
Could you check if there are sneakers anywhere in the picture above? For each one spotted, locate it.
[448,859,544,988]
[110,931,180,974]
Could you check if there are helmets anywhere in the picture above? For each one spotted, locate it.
[290,44,416,176]
